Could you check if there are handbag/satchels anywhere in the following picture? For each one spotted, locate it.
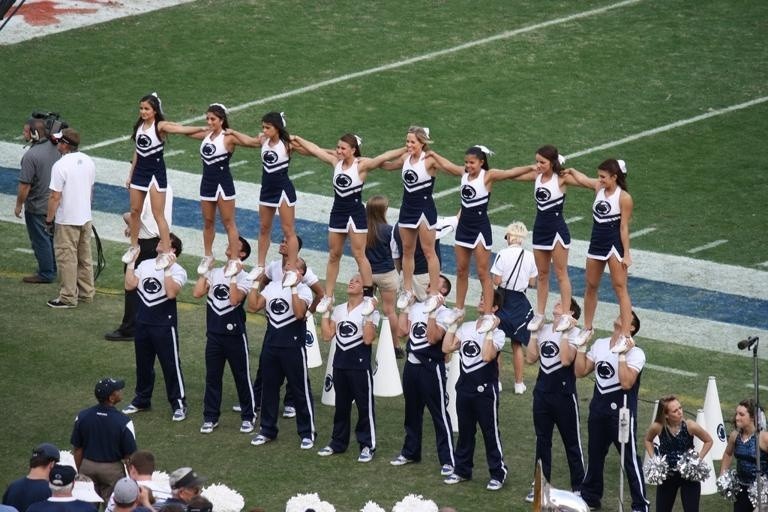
[493,289,505,310]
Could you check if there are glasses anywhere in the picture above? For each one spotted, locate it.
[504,232,509,240]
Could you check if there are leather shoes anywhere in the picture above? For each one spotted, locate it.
[22,277,52,283]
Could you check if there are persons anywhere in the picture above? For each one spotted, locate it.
[489,145,587,332]
[390,208,462,337]
[490,221,538,395]
[290,134,408,317]
[124,235,651,512]
[222,112,337,287]
[646,396,768,511]
[164,102,261,278]
[46,130,96,308]
[0,378,215,511]
[15,119,61,284]
[356,126,458,313]
[561,158,635,353]
[121,91,210,271]
[364,194,405,359]
[420,144,538,333]
[104,186,173,342]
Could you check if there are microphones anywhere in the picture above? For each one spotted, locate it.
[737,335,759,350]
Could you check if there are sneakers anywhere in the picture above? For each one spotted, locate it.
[443,474,468,485]
[283,269,299,287]
[121,244,141,265]
[611,334,635,353]
[395,344,407,359]
[246,266,265,281]
[104,329,135,340]
[283,406,296,418]
[197,251,214,274]
[121,404,148,414]
[155,251,176,271]
[172,407,187,421]
[389,454,414,466]
[300,438,314,449]
[498,381,527,395]
[225,258,243,278]
[487,475,506,490]
[316,292,335,313]
[526,310,546,331]
[441,464,454,476]
[396,286,415,310]
[233,403,271,446]
[478,313,497,334]
[357,446,374,462]
[442,306,465,325]
[525,482,596,510]
[556,313,573,332]
[317,445,335,456]
[571,326,594,346]
[423,293,444,314]
[46,296,94,308]
[200,422,219,433]
[361,294,378,316]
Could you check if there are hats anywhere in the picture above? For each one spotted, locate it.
[52,128,81,146]
[50,465,105,503]
[114,477,139,504]
[33,444,59,462]
[170,467,210,490]
[95,377,125,401]
